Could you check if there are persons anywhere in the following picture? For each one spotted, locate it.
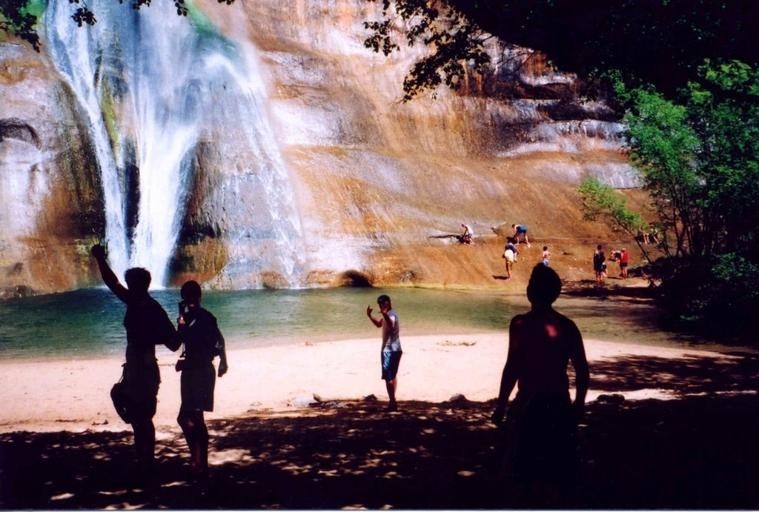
[543,246,549,267]
[462,223,473,245]
[503,243,520,279]
[512,224,528,245]
[594,245,628,281]
[367,295,402,411]
[175,281,228,467]
[91,245,181,474]
[638,223,659,245]
[492,263,589,440]
